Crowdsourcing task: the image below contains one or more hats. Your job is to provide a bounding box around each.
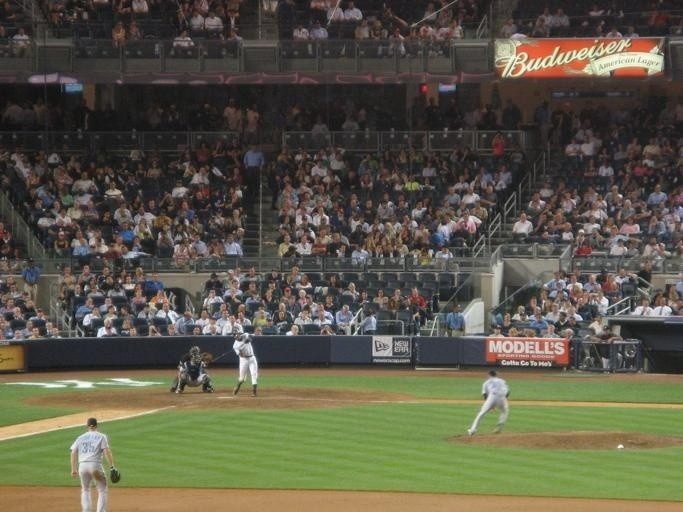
[488,370,496,376]
[87,418,97,427]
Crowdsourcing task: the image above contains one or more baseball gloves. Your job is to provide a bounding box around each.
[109,468,121,483]
[199,351,212,364]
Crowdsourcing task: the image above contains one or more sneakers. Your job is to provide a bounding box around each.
[232,385,256,396]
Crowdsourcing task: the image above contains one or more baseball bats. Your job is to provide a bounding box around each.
[211,340,245,365]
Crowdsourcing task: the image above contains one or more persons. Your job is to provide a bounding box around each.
[230,326,259,396]
[171,345,209,391]
[176,354,214,393]
[465,369,511,435]
[69,418,121,512]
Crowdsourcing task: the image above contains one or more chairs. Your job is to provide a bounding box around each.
[60,271,472,336]
